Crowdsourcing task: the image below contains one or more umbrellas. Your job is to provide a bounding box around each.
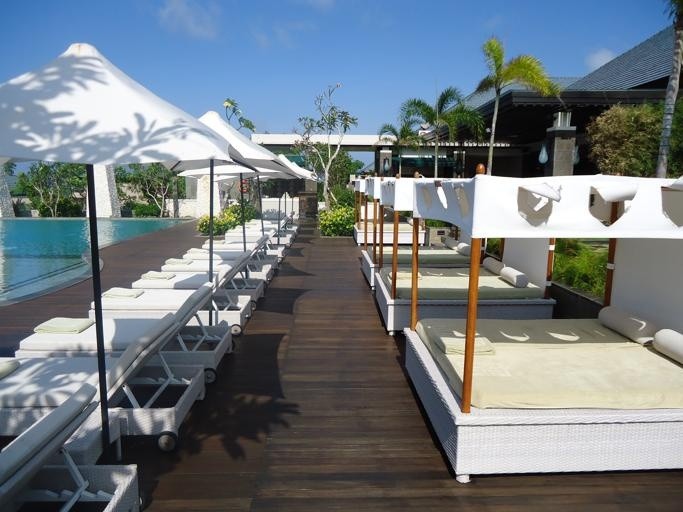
[161,111,297,281]
[0,43,258,455]
[292,161,323,214]
[252,154,312,229]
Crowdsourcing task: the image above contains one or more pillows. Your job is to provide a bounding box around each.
[652,328,682,365]
[483,257,507,275]
[597,304,655,346]
[499,267,529,288]
[457,242,471,256]
[445,237,460,250]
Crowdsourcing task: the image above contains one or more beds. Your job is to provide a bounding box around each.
[354,177,426,246]
[404,164,682,484]
[361,173,487,291]
[375,172,556,336]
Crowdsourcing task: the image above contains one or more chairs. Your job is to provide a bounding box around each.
[14,281,236,384]
[183,247,279,284]
[0,382,140,512]
[201,235,287,265]
[89,263,258,337]
[1,312,206,456]
[131,249,267,311]
[226,211,297,248]
